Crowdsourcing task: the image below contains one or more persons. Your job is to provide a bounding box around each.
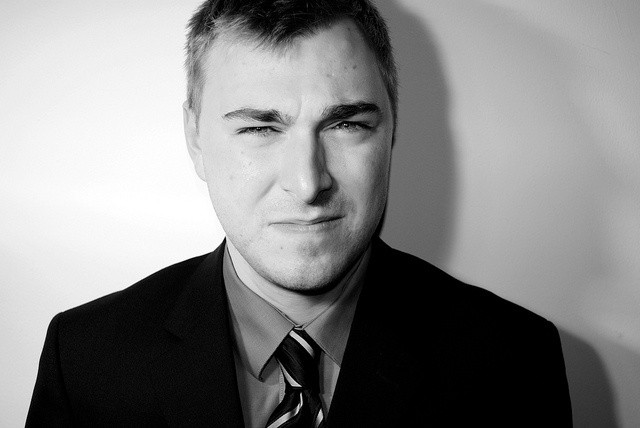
[25,3,573,427]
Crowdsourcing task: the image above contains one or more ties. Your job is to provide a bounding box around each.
[266,328,328,428]
